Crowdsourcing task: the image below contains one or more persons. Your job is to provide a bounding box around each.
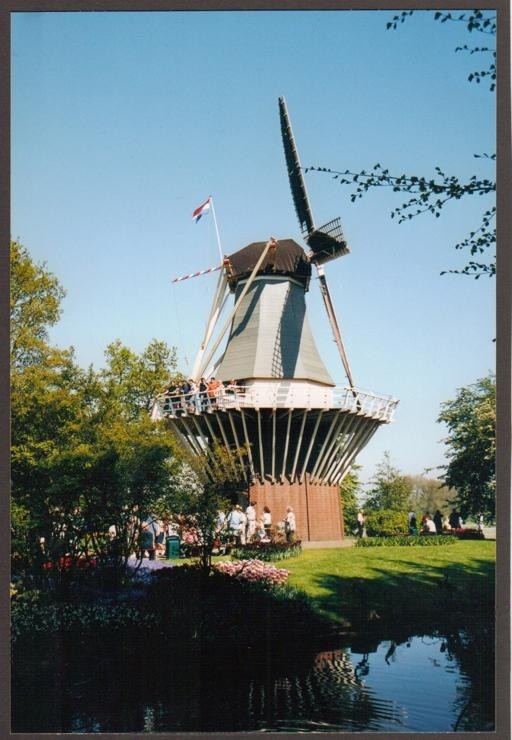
[357,507,369,539]
[408,508,419,535]
[422,512,431,521]
[98,500,299,561]
[423,516,436,535]
[432,509,444,533]
[475,511,484,530]
[448,508,463,530]
[441,515,451,530]
[159,378,245,417]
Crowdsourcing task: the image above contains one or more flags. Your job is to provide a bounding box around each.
[191,196,212,224]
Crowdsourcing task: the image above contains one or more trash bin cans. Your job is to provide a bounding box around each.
[166,535,180,560]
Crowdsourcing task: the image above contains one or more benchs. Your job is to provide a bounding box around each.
[386,533,411,536]
[127,548,159,559]
